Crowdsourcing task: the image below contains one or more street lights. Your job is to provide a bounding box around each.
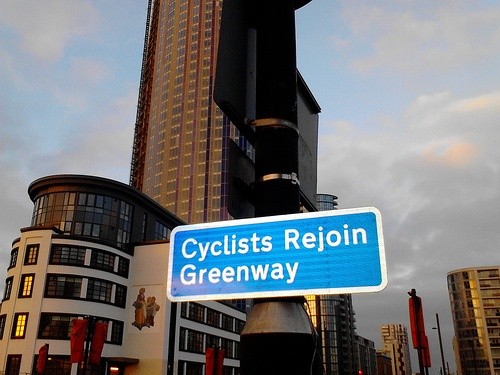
[70,314,108,375]
[203,343,224,375]
[432,313,451,375]
[407,288,431,375]
